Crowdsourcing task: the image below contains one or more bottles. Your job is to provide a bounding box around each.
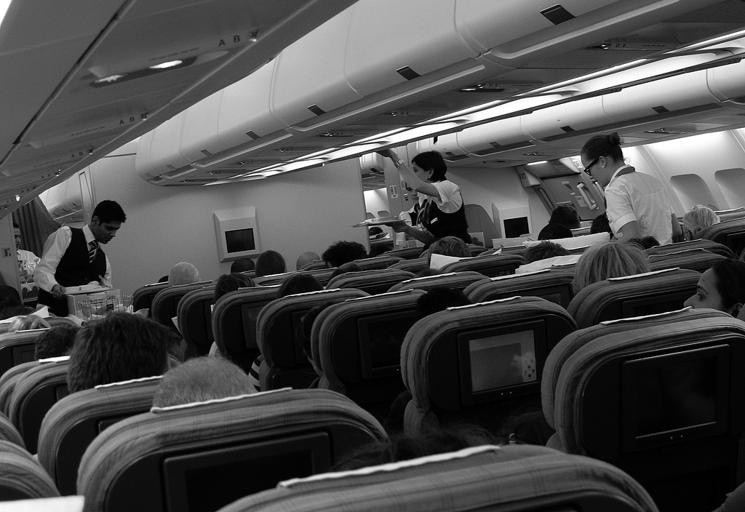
[75,296,119,320]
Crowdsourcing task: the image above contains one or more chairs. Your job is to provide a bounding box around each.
[536,304,744,508]
[209,283,283,375]
[239,284,371,392]
[304,287,433,435]
[73,386,403,512]
[165,288,221,367]
[214,440,659,512]
[2,306,92,502]
[398,295,575,451]
[150,281,202,329]
[227,210,745,330]
[128,276,175,310]
[36,374,181,499]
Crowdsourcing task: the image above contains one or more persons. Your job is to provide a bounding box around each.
[521,201,744,322]
[579,128,686,249]
[14,223,42,278]
[31,197,126,318]
[373,143,472,259]
[1,239,507,475]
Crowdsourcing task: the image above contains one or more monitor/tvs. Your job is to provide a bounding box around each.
[225,228,256,253]
[621,344,732,438]
[241,302,266,349]
[164,432,333,512]
[291,309,312,369]
[458,319,548,406]
[356,310,417,379]
[15,346,34,365]
[504,217,529,238]
[495,286,569,309]
[622,291,697,318]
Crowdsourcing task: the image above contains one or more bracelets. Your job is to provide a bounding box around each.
[393,158,406,170]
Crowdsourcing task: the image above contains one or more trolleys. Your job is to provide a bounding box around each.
[65,281,135,329]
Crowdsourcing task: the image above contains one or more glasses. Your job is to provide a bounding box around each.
[584,160,598,176]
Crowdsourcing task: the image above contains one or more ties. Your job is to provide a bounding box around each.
[88,240,99,264]
